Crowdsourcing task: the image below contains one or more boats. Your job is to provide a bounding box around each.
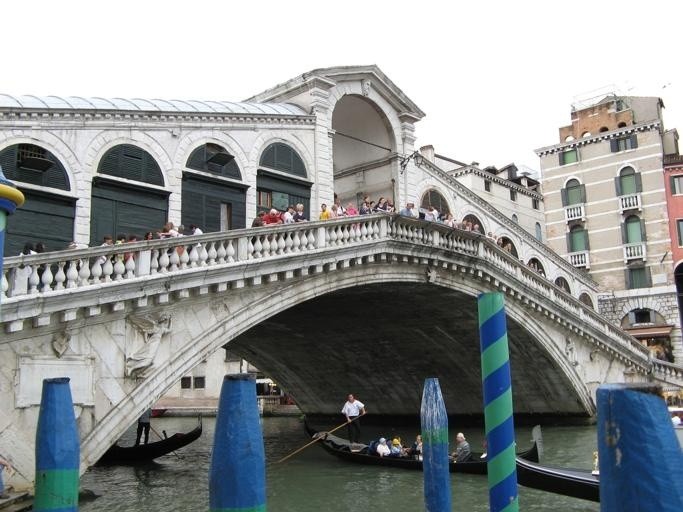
[514,453,600,504]
[303,414,545,473]
[93,413,203,465]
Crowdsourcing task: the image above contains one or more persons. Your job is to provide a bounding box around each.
[247,194,546,278]
[340,392,366,444]
[449,432,471,463]
[363,432,422,465]
[21,221,203,282]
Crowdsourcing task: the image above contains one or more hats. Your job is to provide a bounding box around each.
[379,438,386,443]
[392,439,400,445]
